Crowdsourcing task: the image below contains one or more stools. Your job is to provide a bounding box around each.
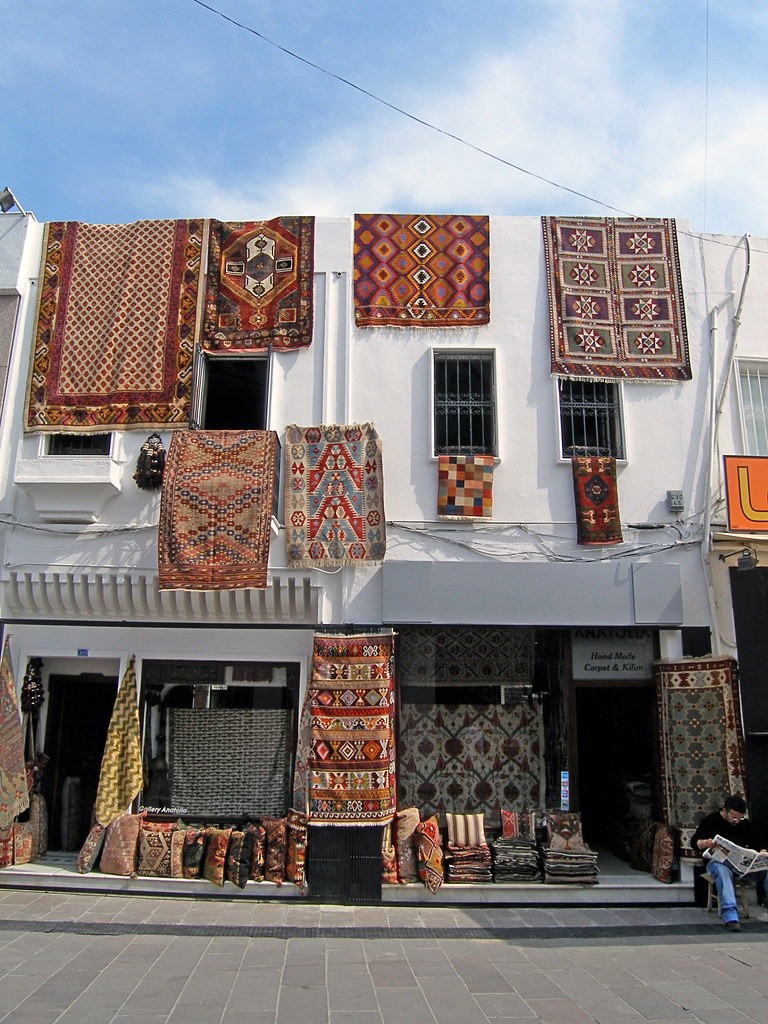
[701,872,747,918]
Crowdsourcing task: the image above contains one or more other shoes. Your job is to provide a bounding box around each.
[726,921,742,931]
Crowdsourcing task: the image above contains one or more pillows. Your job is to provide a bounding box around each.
[546,813,583,849]
[76,806,308,891]
[628,816,656,873]
[380,822,399,885]
[500,809,536,841]
[651,824,673,884]
[444,811,487,846]
[416,813,443,895]
[28,793,49,855]
[395,805,418,885]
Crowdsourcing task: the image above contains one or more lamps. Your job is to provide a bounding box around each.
[718,547,759,572]
[0,186,27,216]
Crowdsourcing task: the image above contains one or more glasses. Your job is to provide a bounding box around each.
[727,812,746,822]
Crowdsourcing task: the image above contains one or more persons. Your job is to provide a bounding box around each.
[690,795,768,931]
